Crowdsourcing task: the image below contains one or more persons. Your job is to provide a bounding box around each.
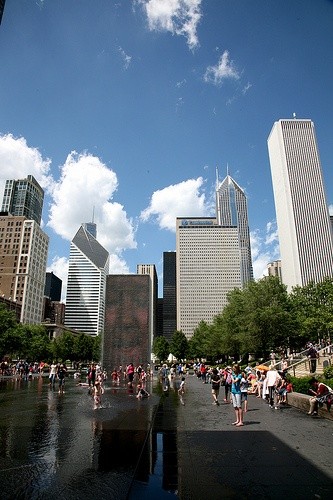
[0,337,333,426]
[210,368,220,406]
